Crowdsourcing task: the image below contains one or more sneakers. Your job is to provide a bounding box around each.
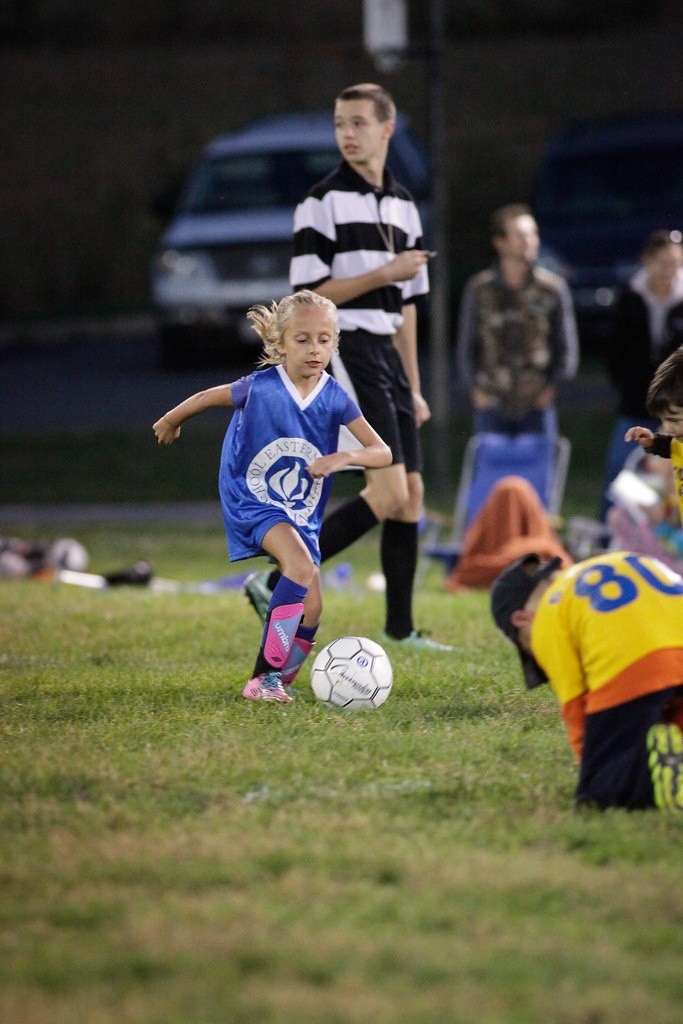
[243,671,294,705]
[242,568,280,624]
[382,628,454,653]
[645,721,683,815]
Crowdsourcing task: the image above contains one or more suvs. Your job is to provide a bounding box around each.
[148,116,430,376]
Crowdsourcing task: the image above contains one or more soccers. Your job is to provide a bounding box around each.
[309,635,395,710]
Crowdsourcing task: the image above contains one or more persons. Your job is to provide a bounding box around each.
[487,549,680,817]
[423,200,582,581]
[582,229,683,558]
[150,289,395,704]
[243,82,460,655]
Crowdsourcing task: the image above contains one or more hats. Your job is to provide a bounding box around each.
[491,552,563,690]
